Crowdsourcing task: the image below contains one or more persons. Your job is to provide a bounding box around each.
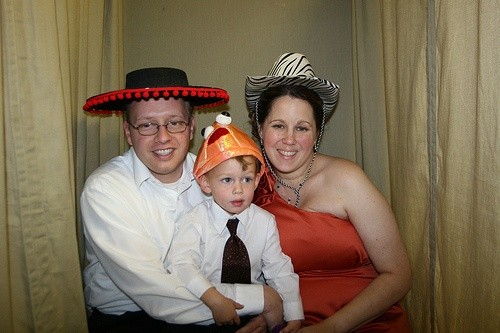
[244,53,414,333]
[79,67,284,333]
[162,112,305,333]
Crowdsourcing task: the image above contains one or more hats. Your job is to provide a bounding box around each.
[82,67,229,116]
[193,112,265,177]
[245,53,339,125]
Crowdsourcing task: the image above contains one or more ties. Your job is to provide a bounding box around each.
[220,217,252,329]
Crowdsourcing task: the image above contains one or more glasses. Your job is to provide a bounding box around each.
[124,110,193,135]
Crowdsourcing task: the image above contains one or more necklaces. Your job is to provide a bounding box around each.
[275,183,302,203]
